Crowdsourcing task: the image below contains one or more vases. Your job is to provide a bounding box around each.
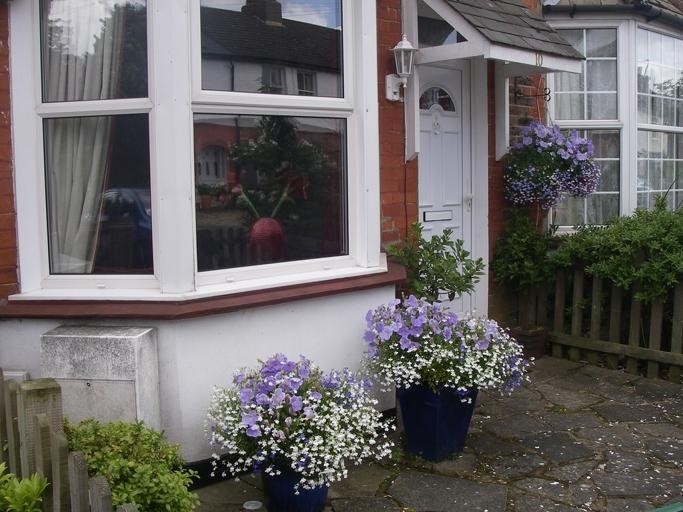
[392,379,480,465]
[246,218,286,263]
[259,454,331,512]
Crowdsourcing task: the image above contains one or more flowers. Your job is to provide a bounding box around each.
[359,294,536,404]
[226,117,332,215]
[500,122,600,210]
[203,351,396,496]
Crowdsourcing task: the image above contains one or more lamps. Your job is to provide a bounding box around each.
[384,33,420,102]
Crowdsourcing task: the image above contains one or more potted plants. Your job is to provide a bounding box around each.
[196,184,221,212]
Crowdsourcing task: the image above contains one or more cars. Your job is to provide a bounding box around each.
[635,176,654,205]
[77,185,215,260]
